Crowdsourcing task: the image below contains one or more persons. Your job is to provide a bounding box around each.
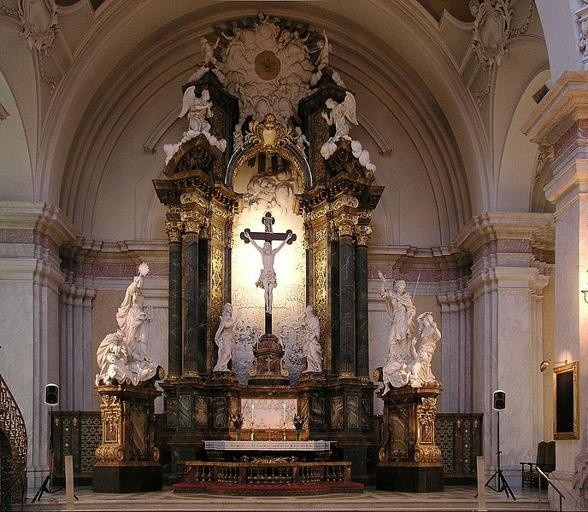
[210,301,240,373]
[242,230,294,316]
[178,10,360,151]
[376,270,441,396]
[93,261,154,388]
[298,304,323,374]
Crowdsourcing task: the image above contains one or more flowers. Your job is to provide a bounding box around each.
[293,413,304,441]
[231,415,244,441]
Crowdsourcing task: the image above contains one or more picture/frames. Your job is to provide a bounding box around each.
[552,361,580,440]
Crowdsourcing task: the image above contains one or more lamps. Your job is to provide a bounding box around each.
[540,359,567,372]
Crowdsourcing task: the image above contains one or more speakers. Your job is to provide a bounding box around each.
[44,383,59,406]
[492,390,506,412]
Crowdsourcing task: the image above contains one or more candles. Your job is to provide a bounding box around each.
[250,405,256,422]
[282,402,285,422]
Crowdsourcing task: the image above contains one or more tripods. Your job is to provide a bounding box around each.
[31,407,78,503]
[474,412,516,501]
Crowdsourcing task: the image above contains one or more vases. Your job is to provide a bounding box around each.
[296,430,302,441]
[236,428,242,441]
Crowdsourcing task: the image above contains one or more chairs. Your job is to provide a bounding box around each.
[520,441,556,489]
